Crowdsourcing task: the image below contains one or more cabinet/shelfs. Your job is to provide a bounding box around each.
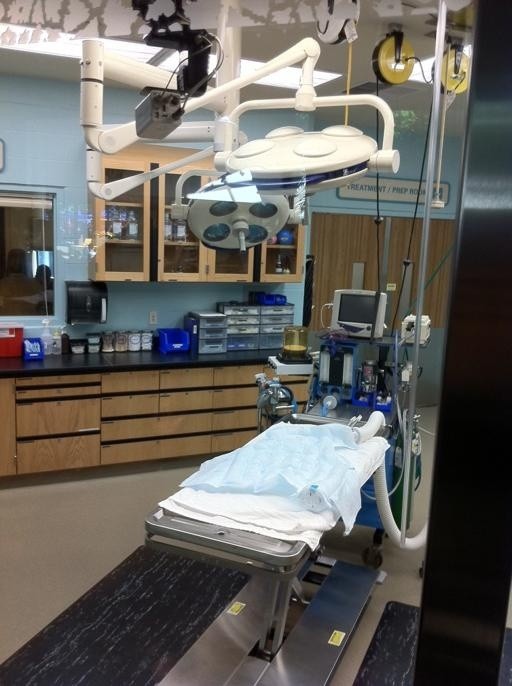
[158,162,255,284]
[16,368,103,489]
[218,303,261,353]
[1,371,17,489]
[215,358,312,456]
[189,309,228,354]
[88,160,154,283]
[256,210,304,285]
[261,303,295,351]
[102,362,215,478]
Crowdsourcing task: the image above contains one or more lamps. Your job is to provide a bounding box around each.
[188,179,289,251]
[228,124,377,193]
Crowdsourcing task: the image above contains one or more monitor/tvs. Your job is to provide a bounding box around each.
[330,288,387,339]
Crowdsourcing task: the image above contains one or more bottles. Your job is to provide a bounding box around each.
[361,359,388,399]
[126,210,138,239]
[276,255,282,273]
[323,392,341,409]
[42,320,153,355]
[112,211,122,239]
[165,213,187,240]
